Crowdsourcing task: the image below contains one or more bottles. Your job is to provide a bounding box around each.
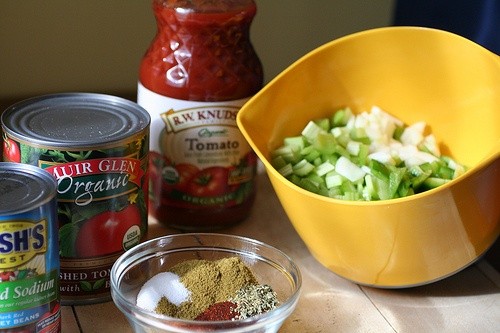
[137,0,264,233]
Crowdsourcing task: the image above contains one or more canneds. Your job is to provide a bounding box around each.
[0,161,63,333]
[0,92,151,305]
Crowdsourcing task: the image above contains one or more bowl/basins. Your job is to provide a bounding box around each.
[110,233,302,333]
[237,26,500,288]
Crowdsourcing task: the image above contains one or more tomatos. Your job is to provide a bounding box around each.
[2,133,21,163]
[145,151,257,203]
[76,205,142,262]
